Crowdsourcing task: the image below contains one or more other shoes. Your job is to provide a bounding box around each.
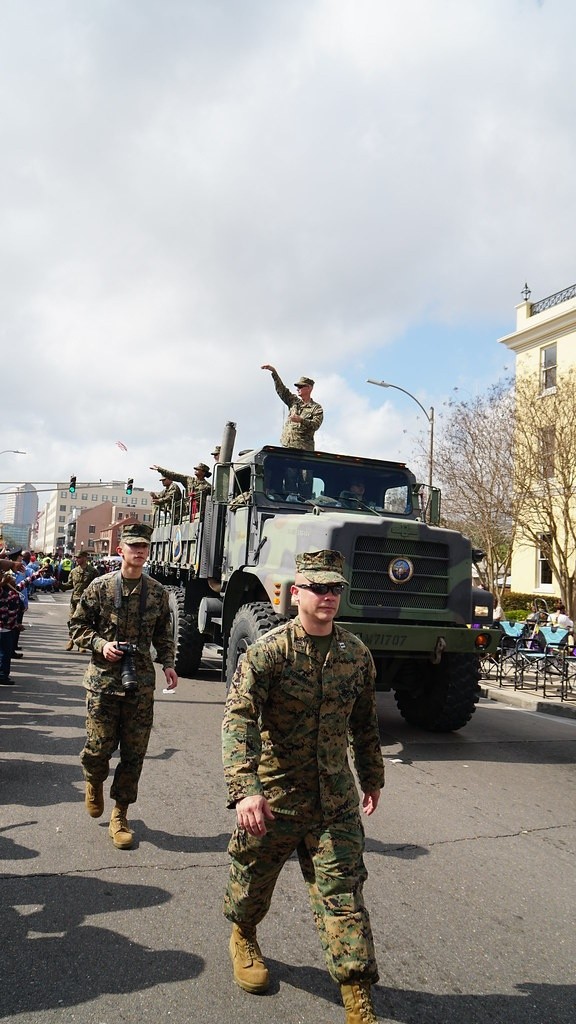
[65,641,73,650]
[15,647,22,650]
[12,653,23,658]
[0,676,15,685]
[78,647,87,652]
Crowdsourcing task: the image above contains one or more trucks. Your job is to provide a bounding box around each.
[132,423,502,733]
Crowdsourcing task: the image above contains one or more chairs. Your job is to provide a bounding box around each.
[479,620,576,703]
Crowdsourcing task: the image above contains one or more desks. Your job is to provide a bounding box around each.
[526,653,576,691]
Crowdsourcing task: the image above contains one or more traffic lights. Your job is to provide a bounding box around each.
[69,476,77,494]
[126,478,134,496]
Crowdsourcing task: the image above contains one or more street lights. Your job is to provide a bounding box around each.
[365,378,436,487]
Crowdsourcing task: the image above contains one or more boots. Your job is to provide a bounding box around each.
[108,801,133,848]
[229,921,269,993]
[86,782,104,818]
[340,979,380,1024]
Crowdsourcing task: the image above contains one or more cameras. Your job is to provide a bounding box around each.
[113,643,141,693]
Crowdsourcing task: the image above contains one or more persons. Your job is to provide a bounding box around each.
[65,550,99,652]
[221,549,385,1024]
[88,559,122,576]
[0,547,78,684]
[149,463,212,512]
[350,478,376,508]
[211,446,221,461]
[472,578,504,629]
[527,604,575,656]
[149,476,186,525]
[71,524,177,847]
[261,364,323,490]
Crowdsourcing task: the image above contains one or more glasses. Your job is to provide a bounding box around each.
[297,584,344,596]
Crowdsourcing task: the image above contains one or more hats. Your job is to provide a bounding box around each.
[295,549,350,586]
[6,546,24,559]
[121,524,153,545]
[294,376,315,386]
[74,549,87,558]
[211,446,221,455]
[159,475,166,481]
[194,462,210,472]
[30,556,36,562]
